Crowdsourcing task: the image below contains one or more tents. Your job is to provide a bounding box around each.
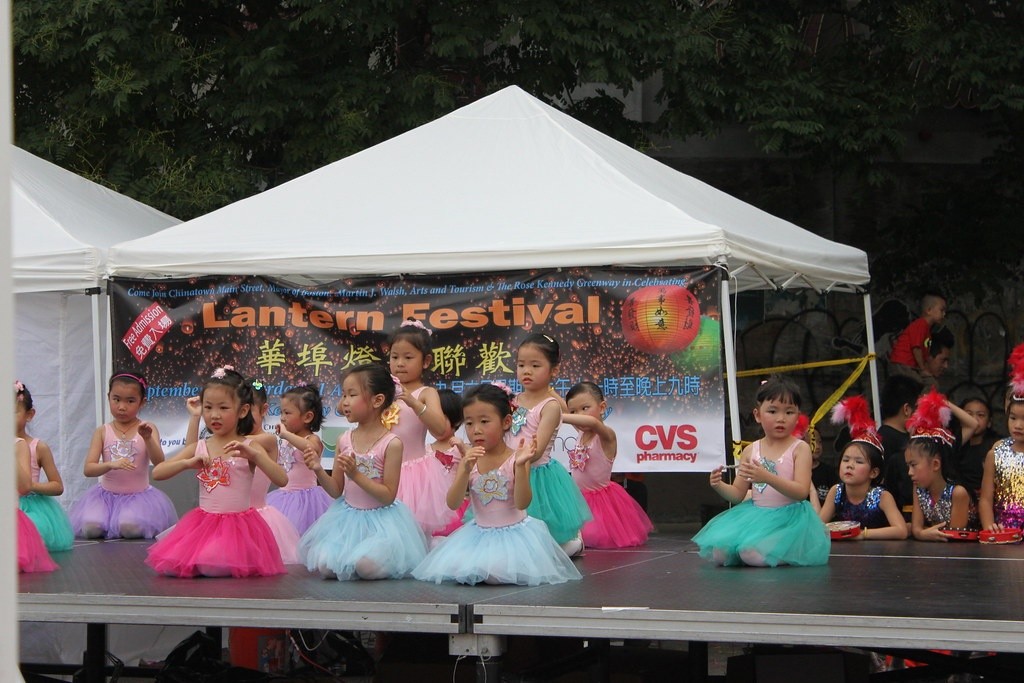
[12,84,883,483]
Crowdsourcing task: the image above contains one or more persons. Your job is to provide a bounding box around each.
[908,426,977,544]
[150,365,300,579]
[68,372,178,539]
[265,386,332,539]
[11,380,74,570]
[294,362,430,582]
[691,376,830,567]
[978,396,1024,532]
[952,397,1005,489]
[815,439,909,542]
[411,383,583,588]
[384,316,460,537]
[503,333,594,557]
[427,389,471,537]
[548,381,654,549]
[876,374,979,505]
[890,295,954,391]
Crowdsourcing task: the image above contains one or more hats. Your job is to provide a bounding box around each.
[904,389,956,448]
[831,395,885,454]
[1005,344,1024,411]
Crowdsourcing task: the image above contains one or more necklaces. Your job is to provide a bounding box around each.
[112,420,139,439]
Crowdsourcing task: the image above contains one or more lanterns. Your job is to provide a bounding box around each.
[620,284,700,358]
[670,316,722,377]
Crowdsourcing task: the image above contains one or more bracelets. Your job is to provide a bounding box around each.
[548,387,554,393]
[418,404,426,418]
[863,527,867,538]
